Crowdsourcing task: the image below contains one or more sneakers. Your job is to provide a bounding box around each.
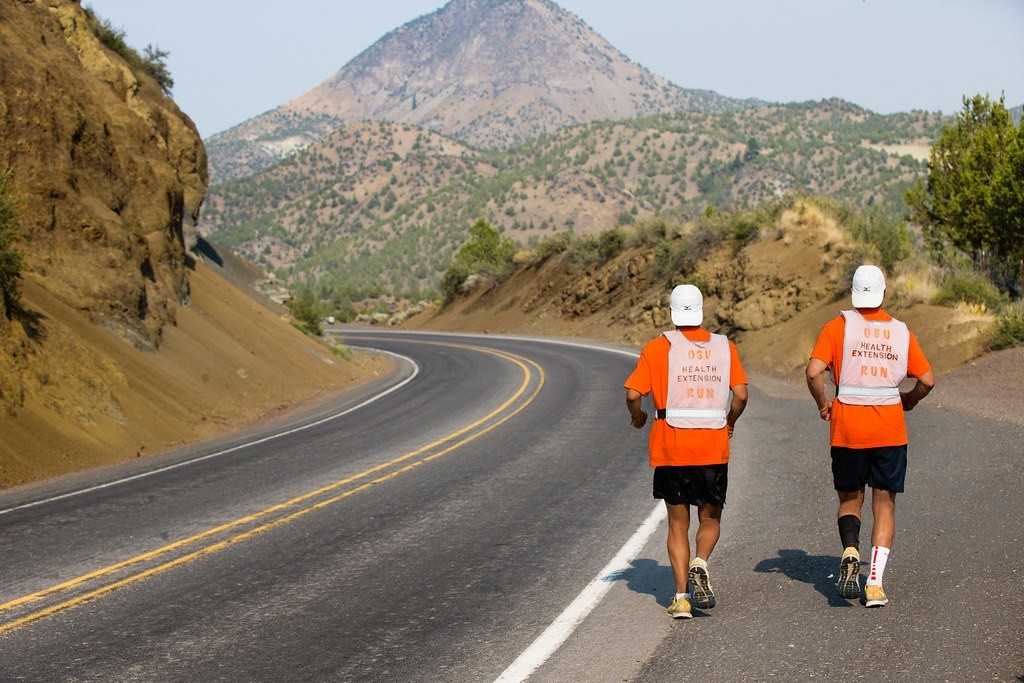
[688,559,716,609]
[837,547,861,600]
[864,584,889,607]
[666,596,693,618]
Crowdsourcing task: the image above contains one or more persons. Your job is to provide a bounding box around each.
[624,284,748,619]
[805,264,935,607]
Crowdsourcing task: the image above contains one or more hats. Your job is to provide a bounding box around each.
[670,285,703,326]
[852,265,886,308]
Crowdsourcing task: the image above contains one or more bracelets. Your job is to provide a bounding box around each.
[818,399,829,412]
[906,393,918,406]
[630,411,645,425]
